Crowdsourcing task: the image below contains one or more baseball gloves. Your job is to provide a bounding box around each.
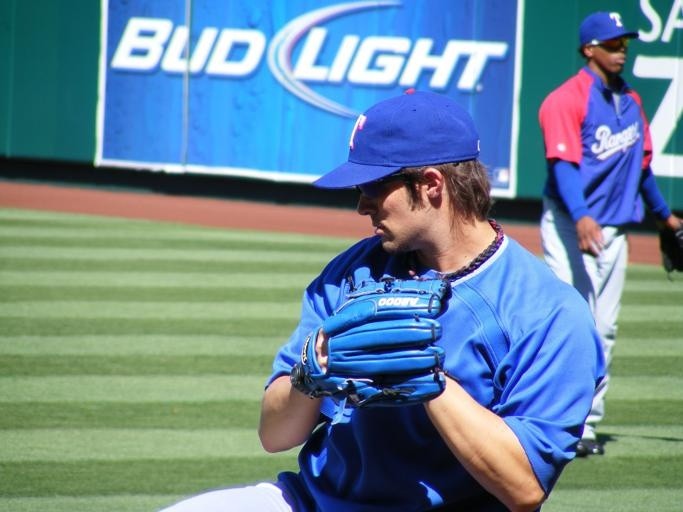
[290,269,452,408]
[657,223,683,280]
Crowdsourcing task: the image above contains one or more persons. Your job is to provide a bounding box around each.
[150,87,606,512]
[534,8,681,459]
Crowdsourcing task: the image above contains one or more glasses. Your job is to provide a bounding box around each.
[580,36,629,52]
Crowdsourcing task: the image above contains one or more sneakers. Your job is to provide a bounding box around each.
[576,438,605,458]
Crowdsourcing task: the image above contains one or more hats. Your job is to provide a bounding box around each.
[311,88,481,191]
[577,10,640,49]
